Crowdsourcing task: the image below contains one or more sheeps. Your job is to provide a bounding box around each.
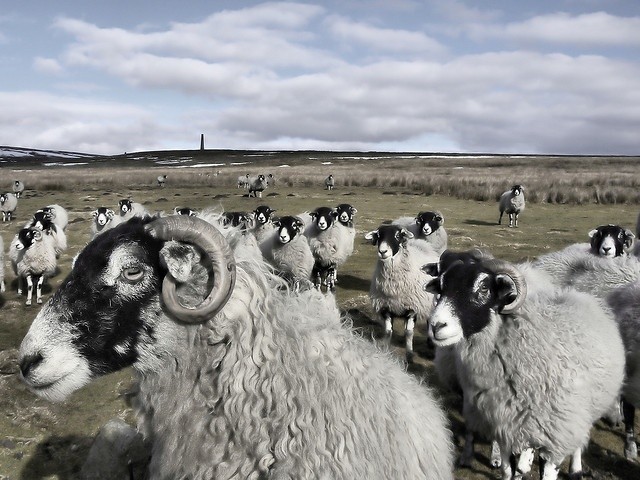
[157,175,167,188]
[18,211,456,479]
[266,173,275,188]
[12,181,24,199]
[531,223,639,299]
[0,192,17,223]
[0,235,6,294]
[89,206,125,234]
[322,204,357,288]
[499,185,525,228]
[249,205,279,260]
[216,210,256,237]
[390,210,446,259]
[420,249,502,467]
[248,175,268,199]
[117,199,148,218]
[257,215,315,291]
[9,227,57,306]
[605,281,639,455]
[296,207,339,294]
[364,223,437,363]
[423,260,627,479]
[237,173,249,189]
[23,212,67,258]
[325,175,334,190]
[36,203,68,232]
